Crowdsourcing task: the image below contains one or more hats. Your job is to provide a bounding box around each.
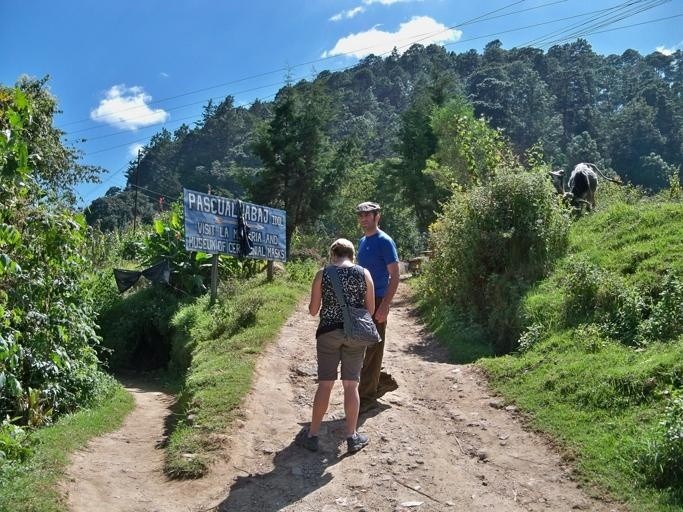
[356,201,381,212]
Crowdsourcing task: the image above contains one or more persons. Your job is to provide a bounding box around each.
[354,201,400,414]
[294,238,376,453]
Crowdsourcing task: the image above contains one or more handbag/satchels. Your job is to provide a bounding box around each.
[343,307,382,345]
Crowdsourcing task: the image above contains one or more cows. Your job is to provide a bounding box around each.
[565,163,623,223]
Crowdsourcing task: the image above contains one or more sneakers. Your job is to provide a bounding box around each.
[295,426,318,451]
[377,377,399,399]
[347,432,369,453]
[360,399,377,413]
